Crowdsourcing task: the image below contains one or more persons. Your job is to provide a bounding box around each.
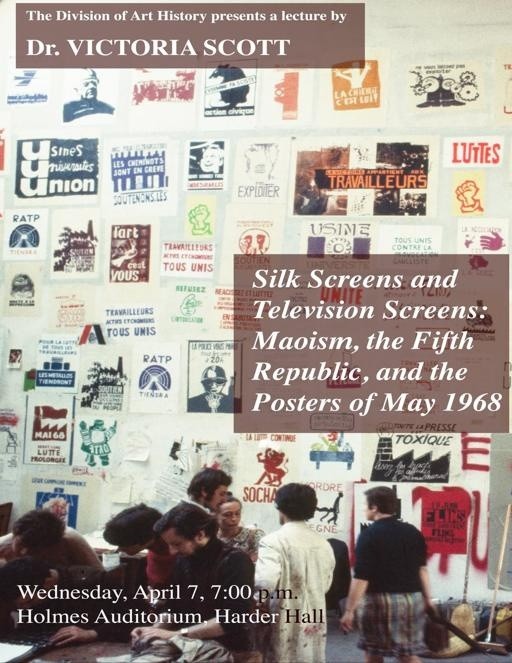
[64,68,114,122]
[188,365,241,412]
[340,487,437,663]
[255,483,336,663]
[0,467,266,649]
[200,144,223,173]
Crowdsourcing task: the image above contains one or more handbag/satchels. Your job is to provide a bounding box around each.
[152,639,235,663]
[423,620,449,651]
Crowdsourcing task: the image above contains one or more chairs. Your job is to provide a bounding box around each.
[325,539,351,635]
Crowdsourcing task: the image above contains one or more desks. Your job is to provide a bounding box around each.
[24,640,264,663]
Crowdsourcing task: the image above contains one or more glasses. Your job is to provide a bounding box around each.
[204,378,228,384]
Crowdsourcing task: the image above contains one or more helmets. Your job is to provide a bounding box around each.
[201,366,227,381]
